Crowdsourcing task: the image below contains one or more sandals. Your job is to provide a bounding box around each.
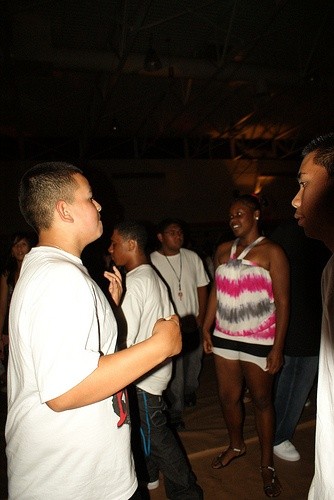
[260,463,283,498]
[211,443,247,470]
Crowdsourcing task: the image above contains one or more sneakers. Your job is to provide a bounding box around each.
[273,439,301,463]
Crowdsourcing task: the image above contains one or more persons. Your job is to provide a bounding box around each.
[203,193,289,498]
[0,135,334,500]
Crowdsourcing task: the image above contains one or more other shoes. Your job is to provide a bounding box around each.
[147,480,160,490]
[185,393,197,406]
[170,413,185,432]
